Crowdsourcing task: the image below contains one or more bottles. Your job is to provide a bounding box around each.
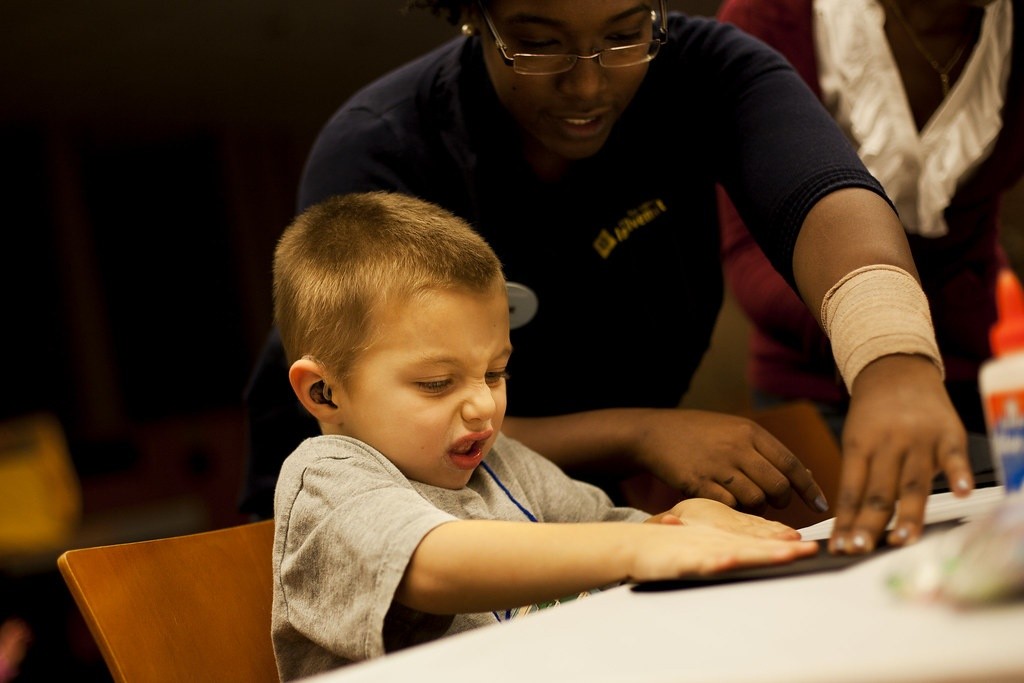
[975,268,1024,497]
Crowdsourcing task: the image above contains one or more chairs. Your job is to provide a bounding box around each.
[55,518,325,683]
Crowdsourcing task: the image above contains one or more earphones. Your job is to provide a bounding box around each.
[310,381,338,407]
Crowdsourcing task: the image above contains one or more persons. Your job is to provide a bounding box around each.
[707,0,1024,491]
[238,1,972,556]
[272,193,819,683]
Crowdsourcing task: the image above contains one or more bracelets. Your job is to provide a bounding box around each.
[820,265,946,398]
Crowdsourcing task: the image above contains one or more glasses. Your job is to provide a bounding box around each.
[474,1,669,76]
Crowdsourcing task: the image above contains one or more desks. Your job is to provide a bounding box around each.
[268,485,1023,679]
